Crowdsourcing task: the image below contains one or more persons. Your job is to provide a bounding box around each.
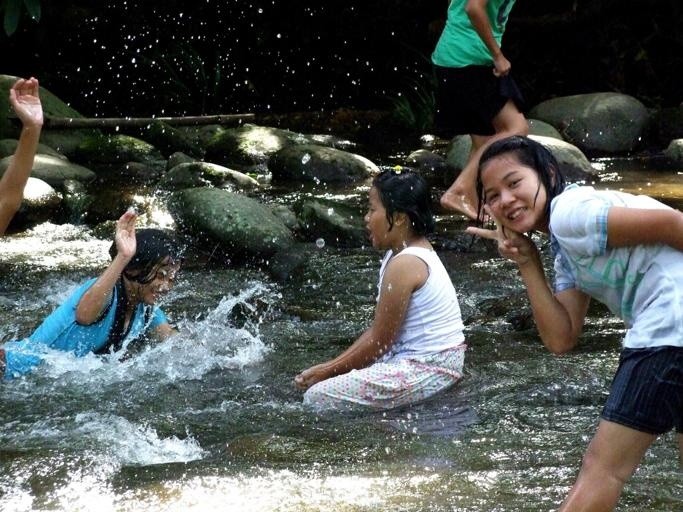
[466,134,683,512]
[294,169,468,421]
[0,76,44,236]
[431,0,529,222]
[0,209,188,383]
